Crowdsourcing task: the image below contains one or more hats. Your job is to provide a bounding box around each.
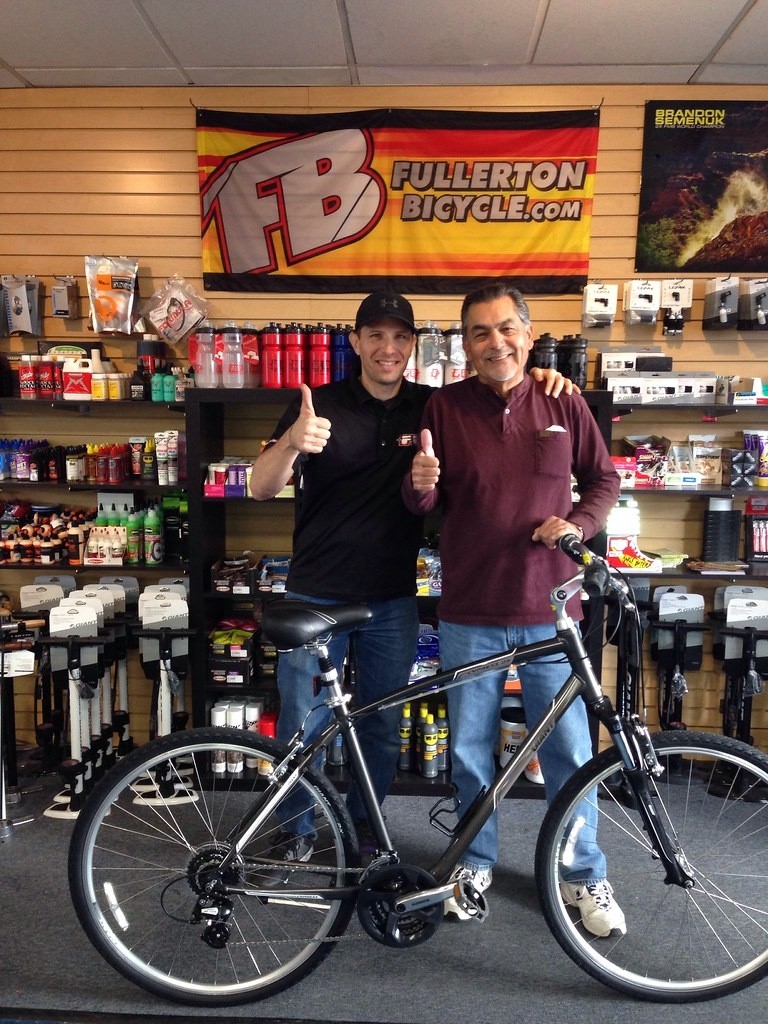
[355,293,417,333]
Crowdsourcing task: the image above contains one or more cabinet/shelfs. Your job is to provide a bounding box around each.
[182,385,614,800]
[608,398,768,580]
[0,394,185,575]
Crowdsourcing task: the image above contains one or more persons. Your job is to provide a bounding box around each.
[249,290,582,890]
[400,283,627,937]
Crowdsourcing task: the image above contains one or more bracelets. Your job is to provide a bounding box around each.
[575,524,584,542]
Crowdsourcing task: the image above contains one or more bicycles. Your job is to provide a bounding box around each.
[68,519,768,1009]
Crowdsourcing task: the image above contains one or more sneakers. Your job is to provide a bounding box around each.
[250,832,313,889]
[558,881,626,937]
[444,866,493,920]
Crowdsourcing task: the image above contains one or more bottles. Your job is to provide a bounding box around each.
[0,318,589,789]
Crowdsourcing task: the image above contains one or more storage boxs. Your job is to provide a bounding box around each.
[202,480,444,690]
